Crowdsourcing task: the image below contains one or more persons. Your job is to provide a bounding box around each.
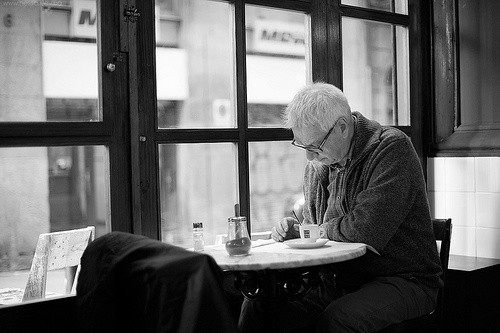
[238,82,446,333]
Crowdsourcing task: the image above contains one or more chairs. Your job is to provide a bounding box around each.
[67,232,208,333]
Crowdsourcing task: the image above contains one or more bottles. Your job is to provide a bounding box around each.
[225,217,250,256]
[192,222,205,252]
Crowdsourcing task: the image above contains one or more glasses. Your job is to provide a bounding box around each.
[291,117,347,153]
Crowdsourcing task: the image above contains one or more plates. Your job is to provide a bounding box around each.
[283,238,329,248]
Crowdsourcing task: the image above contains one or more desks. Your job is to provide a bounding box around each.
[180,235,368,333]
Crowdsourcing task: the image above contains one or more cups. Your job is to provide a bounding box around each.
[299,225,325,239]
